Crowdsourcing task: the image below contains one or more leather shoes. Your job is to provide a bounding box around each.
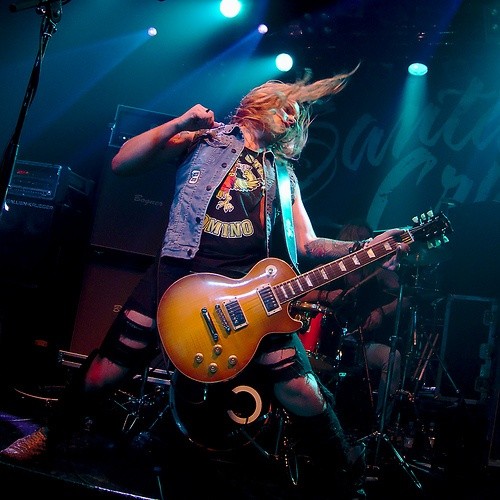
[0,426,47,460]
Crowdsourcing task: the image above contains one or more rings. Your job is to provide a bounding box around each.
[206,109,211,113]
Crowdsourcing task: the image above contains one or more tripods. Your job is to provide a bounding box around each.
[349,251,421,490]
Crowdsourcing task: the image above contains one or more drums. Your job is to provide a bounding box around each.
[288,301,347,374]
[170,370,270,451]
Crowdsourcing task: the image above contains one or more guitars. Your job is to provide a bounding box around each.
[156,209,456,384]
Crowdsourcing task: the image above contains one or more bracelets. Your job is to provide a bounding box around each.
[378,305,387,322]
[349,239,366,256]
[325,290,331,303]
[317,290,322,302]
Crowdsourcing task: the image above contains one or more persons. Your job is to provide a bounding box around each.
[0,61,411,500]
[308,219,414,448]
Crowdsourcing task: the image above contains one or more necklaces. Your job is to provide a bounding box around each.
[234,134,271,164]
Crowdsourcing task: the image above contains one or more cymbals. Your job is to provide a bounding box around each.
[383,287,439,299]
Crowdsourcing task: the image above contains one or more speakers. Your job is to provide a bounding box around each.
[90,146,183,258]
[414,294,499,405]
